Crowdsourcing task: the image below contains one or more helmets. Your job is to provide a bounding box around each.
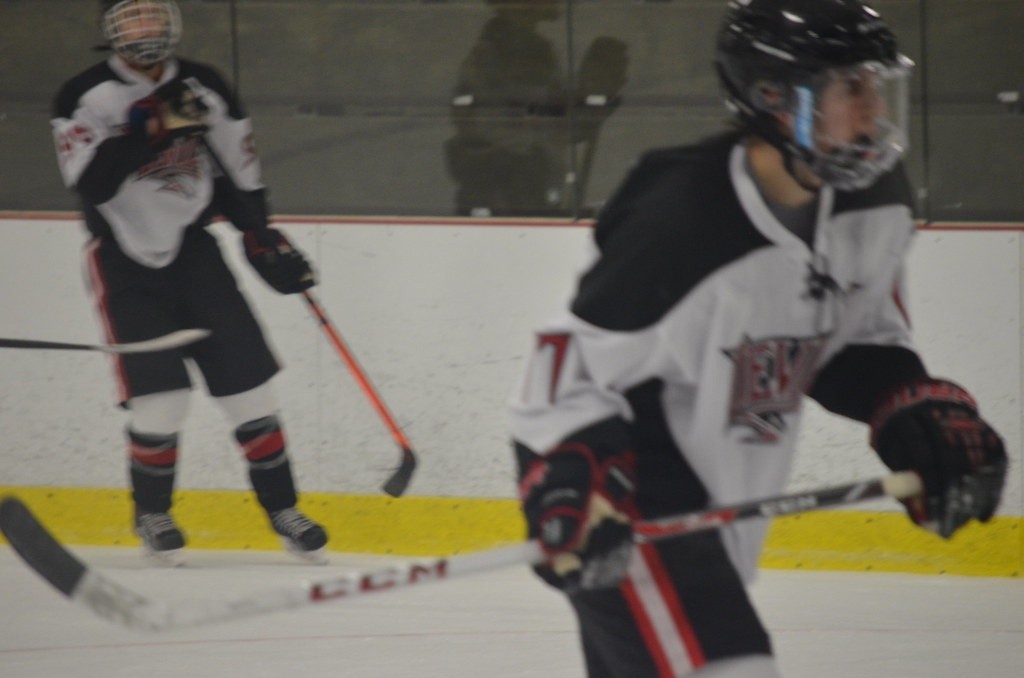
[716,0,900,191]
[93,0,182,70]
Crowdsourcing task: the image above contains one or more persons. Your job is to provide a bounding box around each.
[48,0,330,553]
[444,0,630,219]
[509,0,1011,678]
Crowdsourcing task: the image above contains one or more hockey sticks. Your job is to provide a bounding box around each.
[0,328,215,354]
[197,133,418,499]
[571,133,594,218]
[0,472,925,636]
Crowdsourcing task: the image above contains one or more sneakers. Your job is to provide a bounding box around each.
[134,505,186,569]
[268,508,331,567]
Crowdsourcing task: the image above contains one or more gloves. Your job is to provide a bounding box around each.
[870,378,1008,542]
[129,80,212,151]
[514,420,639,599]
[241,224,318,294]
